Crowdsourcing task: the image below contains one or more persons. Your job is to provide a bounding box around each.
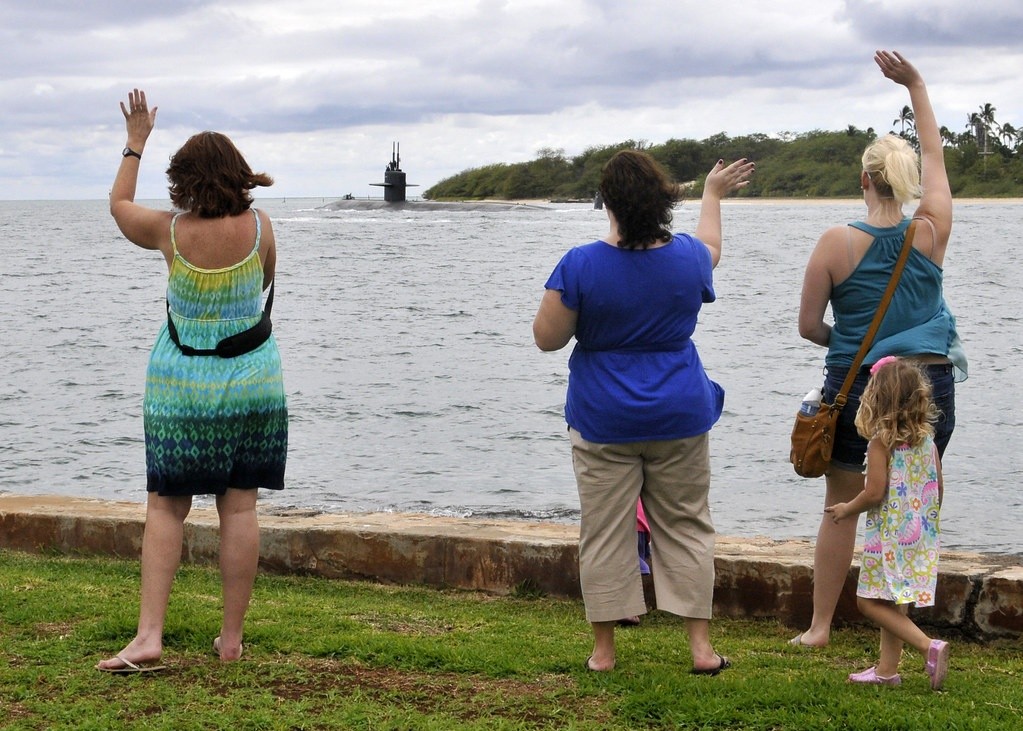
[616,494,650,626]
[823,355,951,691]
[786,50,956,668]
[93,87,289,673]
[532,148,756,677]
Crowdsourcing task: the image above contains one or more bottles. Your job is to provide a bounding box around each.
[801,387,823,417]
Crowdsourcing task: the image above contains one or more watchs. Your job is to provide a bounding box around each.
[122,146,142,160]
